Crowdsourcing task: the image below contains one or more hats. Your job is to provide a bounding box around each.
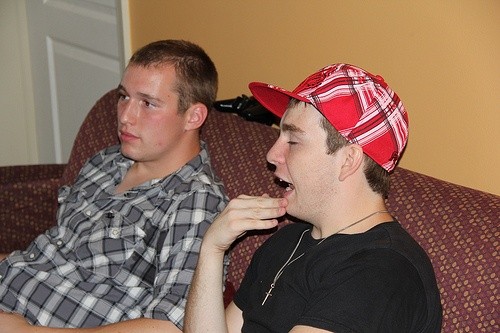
[247,62,411,172]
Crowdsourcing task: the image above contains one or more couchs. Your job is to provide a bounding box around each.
[0,86,500,333]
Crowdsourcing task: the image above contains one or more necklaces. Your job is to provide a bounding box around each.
[261,210,389,306]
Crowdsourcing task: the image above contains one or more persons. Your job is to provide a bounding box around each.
[0,39,230,333]
[183,63,442,333]
